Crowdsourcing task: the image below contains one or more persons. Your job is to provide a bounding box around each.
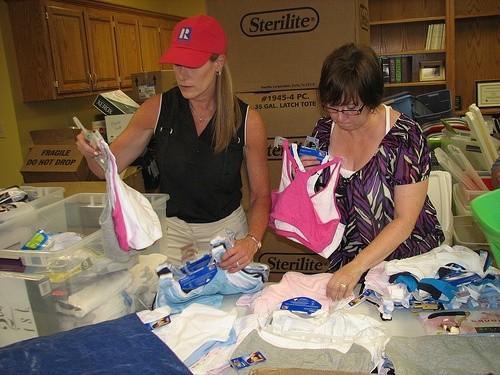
[292,43,445,302]
[76,14,271,273]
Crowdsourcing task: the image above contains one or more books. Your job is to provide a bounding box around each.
[377,54,412,84]
[425,24,446,50]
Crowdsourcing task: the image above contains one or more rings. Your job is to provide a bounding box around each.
[341,283,348,287]
[237,261,240,267]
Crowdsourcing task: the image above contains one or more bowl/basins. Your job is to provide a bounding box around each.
[468,187,500,270]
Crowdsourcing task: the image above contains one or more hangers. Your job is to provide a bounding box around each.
[72,114,109,155]
[273,129,345,170]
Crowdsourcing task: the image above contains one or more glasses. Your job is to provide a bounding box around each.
[320,102,366,116]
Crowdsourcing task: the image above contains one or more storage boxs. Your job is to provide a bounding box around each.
[0,69,176,347]
[203,0,500,281]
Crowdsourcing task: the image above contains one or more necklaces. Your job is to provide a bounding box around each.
[190,104,203,122]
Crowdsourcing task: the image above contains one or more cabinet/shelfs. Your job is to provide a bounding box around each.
[0,0,196,102]
[368,0,500,123]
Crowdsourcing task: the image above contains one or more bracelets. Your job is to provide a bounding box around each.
[247,234,262,249]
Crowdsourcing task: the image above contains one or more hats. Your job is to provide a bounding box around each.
[158,14,227,68]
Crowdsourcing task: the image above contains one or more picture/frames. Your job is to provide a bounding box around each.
[418,59,445,81]
